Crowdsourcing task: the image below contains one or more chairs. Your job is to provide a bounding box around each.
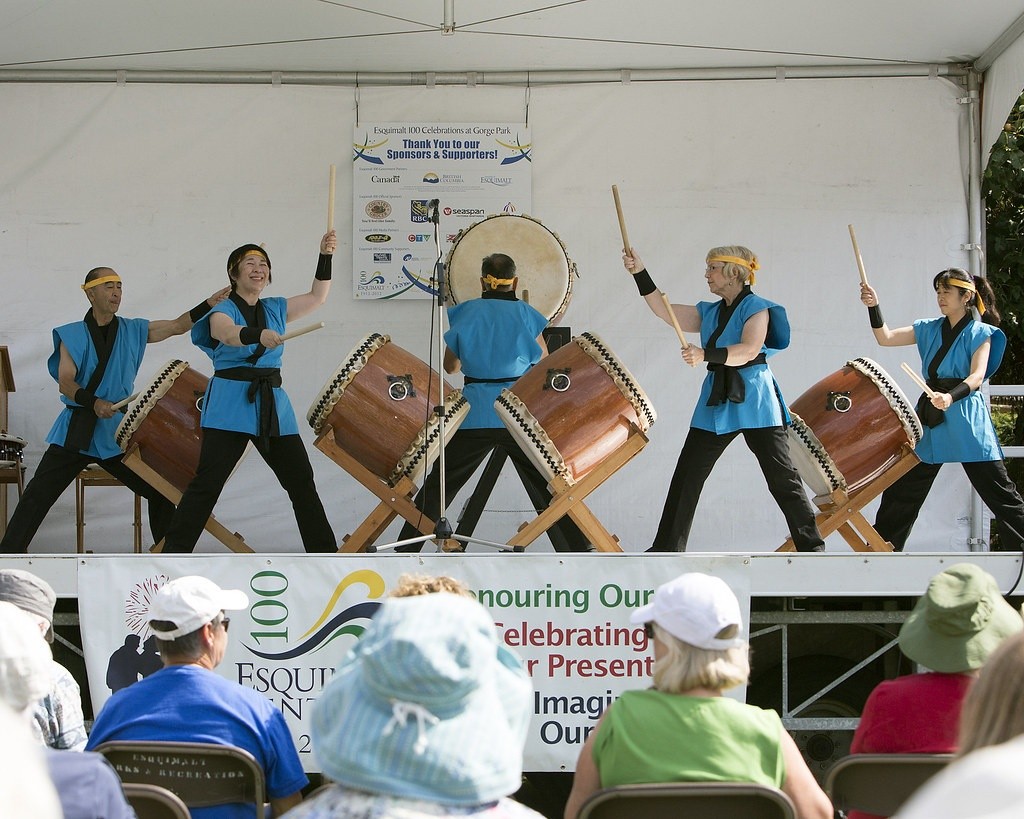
[89,740,959,818]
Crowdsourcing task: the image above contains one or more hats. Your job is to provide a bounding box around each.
[310,593,536,807]
[630,568,741,651]
[897,564,1024,673]
[0,602,53,725]
[0,568,57,644]
[146,575,250,641]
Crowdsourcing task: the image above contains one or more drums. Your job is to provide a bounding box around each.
[443,210,574,328]
[303,329,472,487]
[783,353,925,499]
[492,328,657,486]
[0,432,28,469]
[112,358,211,493]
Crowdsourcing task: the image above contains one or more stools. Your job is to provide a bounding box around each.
[75,468,142,555]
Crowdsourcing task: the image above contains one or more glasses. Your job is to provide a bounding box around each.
[645,621,656,639]
[705,265,724,273]
[215,618,230,633]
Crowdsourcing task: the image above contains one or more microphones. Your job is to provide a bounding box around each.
[427,199,440,209]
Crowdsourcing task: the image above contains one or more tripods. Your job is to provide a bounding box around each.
[366,203,526,556]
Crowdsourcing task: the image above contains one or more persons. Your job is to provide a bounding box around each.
[84,576,310,819]
[859,267,1024,552]
[564,572,833,819]
[847,563,1023,819]
[0,267,232,553]
[161,230,339,553]
[276,574,547,818]
[0,568,138,819]
[890,631,1024,819]
[394,253,598,554]
[622,246,825,553]
